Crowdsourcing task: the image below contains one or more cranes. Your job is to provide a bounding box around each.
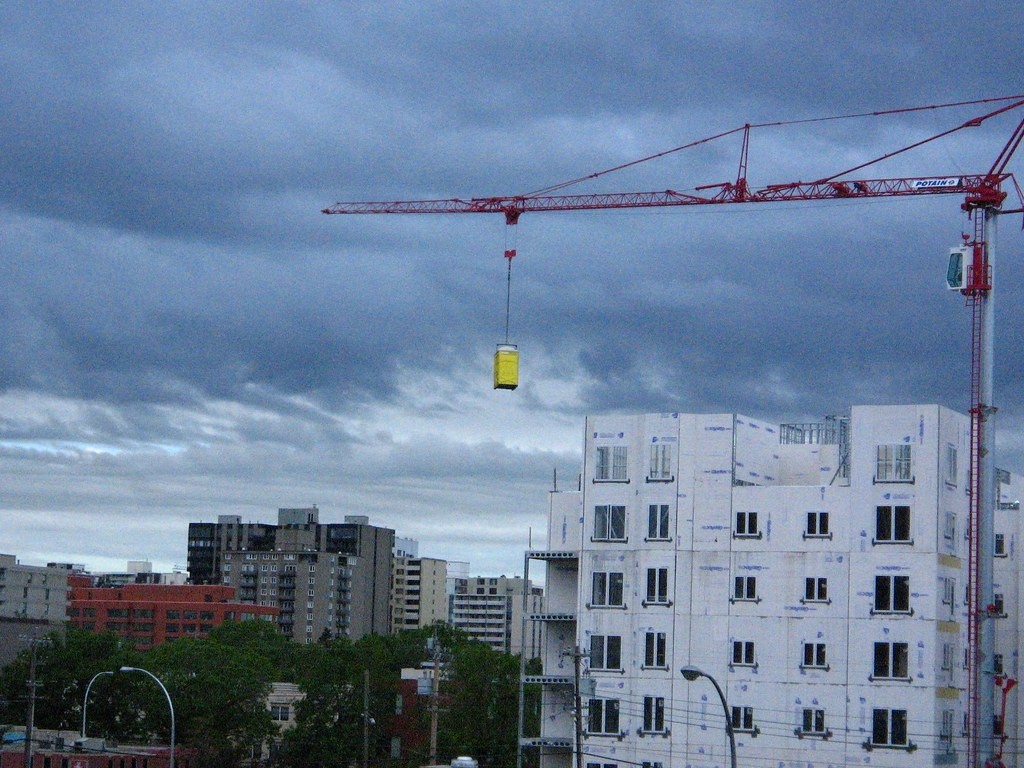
[320,90,1023,766]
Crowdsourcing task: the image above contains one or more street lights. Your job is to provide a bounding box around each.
[81,671,113,737]
[120,665,176,768]
[10,626,53,767]
[678,665,737,768]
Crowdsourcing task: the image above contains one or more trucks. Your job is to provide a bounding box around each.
[69,735,108,752]
